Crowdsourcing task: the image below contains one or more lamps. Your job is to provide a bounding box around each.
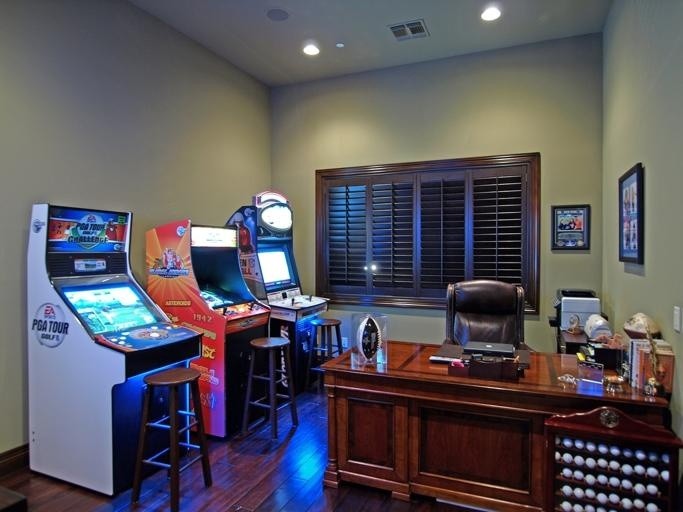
[623,312,661,396]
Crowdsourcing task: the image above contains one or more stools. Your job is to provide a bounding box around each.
[132,368,213,511]
[309,318,343,394]
[242,337,299,439]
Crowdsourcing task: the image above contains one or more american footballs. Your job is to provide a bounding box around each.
[354,317,382,361]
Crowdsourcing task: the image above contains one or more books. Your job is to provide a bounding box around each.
[429,345,464,362]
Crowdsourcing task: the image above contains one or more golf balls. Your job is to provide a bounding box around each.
[555,437,669,511]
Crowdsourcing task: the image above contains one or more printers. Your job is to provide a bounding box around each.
[549,296,601,331]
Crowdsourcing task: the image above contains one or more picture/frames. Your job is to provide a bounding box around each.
[551,205,590,250]
[617,161,645,265]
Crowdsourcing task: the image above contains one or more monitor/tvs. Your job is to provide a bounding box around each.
[258,247,291,287]
[62,282,161,335]
[199,286,235,308]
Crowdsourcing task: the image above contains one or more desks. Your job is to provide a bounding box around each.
[321,339,672,511]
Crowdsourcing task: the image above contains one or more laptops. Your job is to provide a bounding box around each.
[464,341,514,357]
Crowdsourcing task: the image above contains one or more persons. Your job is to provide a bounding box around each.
[559,216,583,230]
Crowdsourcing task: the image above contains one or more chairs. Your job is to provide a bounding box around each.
[543,404,683,510]
[442,280,533,351]
